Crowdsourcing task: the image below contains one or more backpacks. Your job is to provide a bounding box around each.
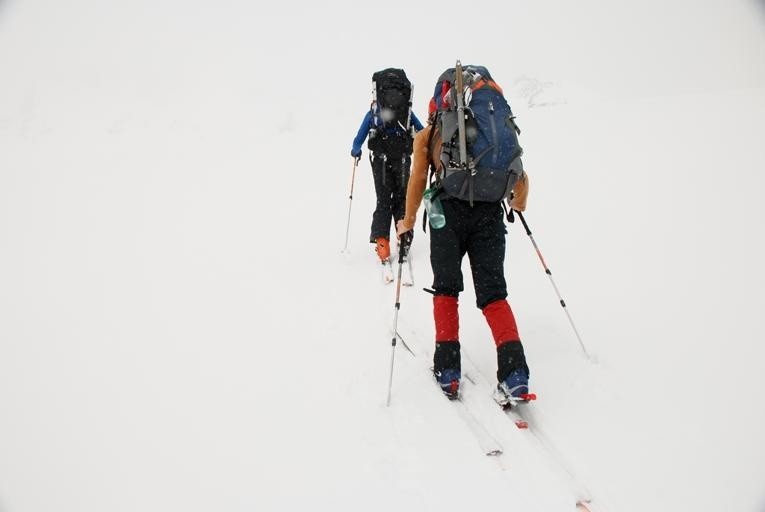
[371,68,414,160]
[433,65,523,202]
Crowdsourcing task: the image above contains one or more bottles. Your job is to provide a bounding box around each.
[423,188,448,231]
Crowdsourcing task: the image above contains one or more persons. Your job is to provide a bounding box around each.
[390,97,535,406]
[350,92,426,265]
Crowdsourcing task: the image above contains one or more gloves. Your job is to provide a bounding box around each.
[351,149,361,157]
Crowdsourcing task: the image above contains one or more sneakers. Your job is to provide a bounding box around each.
[495,370,530,405]
[436,368,461,400]
[376,237,391,264]
